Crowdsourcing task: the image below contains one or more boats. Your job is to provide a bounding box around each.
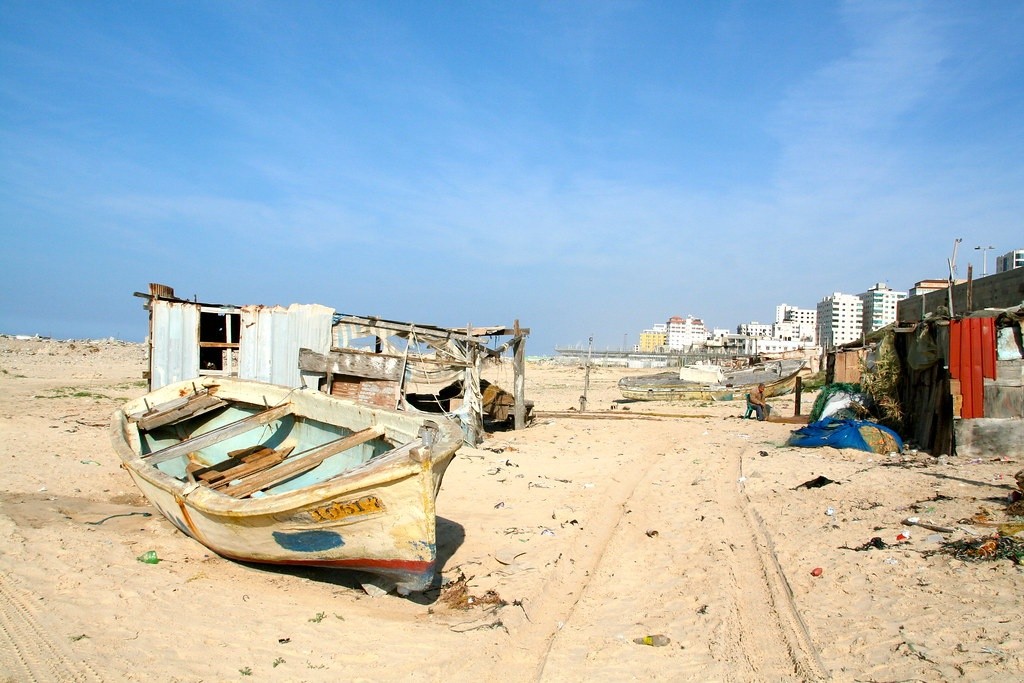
[618,357,808,401]
[785,420,899,454]
[109,375,464,592]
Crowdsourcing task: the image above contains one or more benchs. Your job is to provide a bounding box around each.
[140,403,295,466]
[220,424,386,499]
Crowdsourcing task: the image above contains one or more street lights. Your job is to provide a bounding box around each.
[975,245,995,277]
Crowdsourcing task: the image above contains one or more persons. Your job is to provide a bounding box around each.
[749,383,766,421]
